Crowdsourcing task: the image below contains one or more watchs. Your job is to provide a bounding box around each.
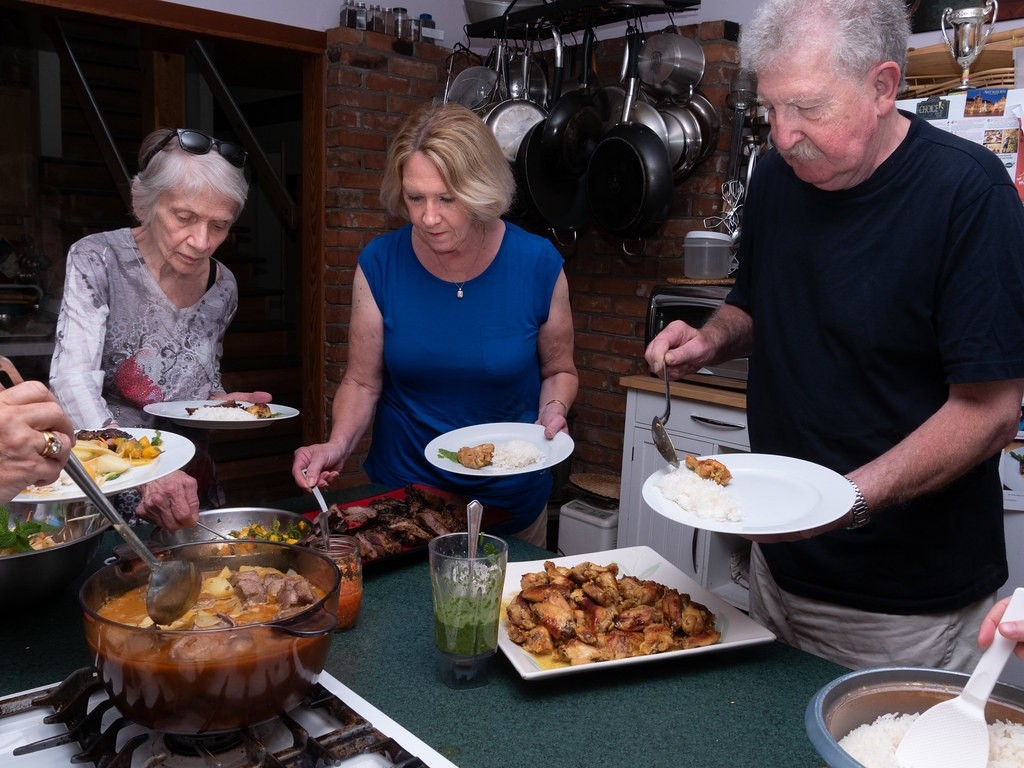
[844,476,871,530]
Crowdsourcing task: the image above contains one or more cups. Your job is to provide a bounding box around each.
[428,530,508,688]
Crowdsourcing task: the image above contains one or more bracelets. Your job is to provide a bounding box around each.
[546,400,568,418]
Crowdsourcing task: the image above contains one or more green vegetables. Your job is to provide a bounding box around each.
[230,515,302,543]
[438,449,458,463]
[0,505,55,553]
[105,429,163,480]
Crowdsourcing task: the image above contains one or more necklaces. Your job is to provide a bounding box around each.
[436,224,486,298]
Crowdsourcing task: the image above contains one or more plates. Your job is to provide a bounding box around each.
[142,396,301,426]
[638,453,860,535]
[422,421,577,478]
[8,431,194,502]
[496,545,778,679]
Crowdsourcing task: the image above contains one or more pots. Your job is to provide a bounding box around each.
[444,22,721,267]
[807,666,1024,768]
[75,538,343,735]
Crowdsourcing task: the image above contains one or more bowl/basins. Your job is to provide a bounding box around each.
[150,507,314,564]
[0,503,114,596]
[681,230,732,278]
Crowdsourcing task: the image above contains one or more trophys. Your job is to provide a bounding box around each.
[941,0,998,90]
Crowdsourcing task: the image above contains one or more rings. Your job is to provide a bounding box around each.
[40,430,62,457]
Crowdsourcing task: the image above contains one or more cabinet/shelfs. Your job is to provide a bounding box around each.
[617,388,1024,691]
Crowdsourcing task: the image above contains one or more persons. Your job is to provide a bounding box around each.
[49,129,273,531]
[645,0,1024,675]
[0,380,76,507]
[978,595,1024,660]
[292,103,579,550]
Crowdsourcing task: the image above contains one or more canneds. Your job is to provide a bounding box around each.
[311,534,363,632]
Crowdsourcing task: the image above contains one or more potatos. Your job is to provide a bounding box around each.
[201,565,285,597]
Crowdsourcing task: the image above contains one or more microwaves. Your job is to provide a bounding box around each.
[646,283,750,392]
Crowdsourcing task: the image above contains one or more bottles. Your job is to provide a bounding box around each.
[299,534,363,636]
[340,1,440,45]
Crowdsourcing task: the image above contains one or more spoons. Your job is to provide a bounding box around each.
[651,358,681,468]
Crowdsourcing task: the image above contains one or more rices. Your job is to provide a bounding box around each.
[189,406,257,421]
[492,440,549,468]
[837,712,1024,768]
[652,467,743,523]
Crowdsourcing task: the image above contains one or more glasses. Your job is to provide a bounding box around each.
[142,129,249,170]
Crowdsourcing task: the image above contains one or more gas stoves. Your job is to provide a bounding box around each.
[0,667,459,768]
